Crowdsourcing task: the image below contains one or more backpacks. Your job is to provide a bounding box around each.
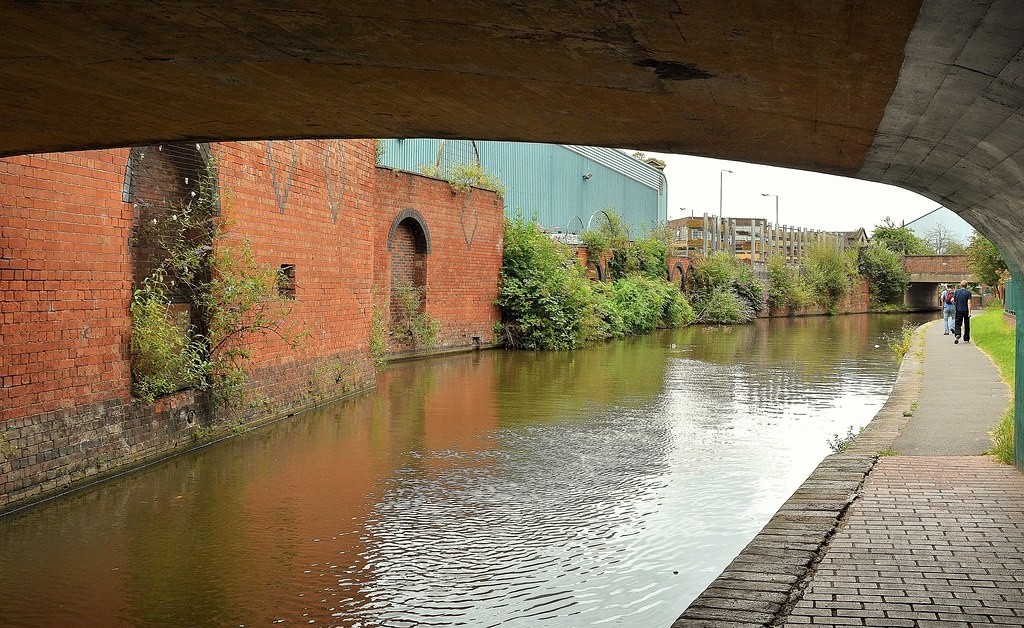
[945,289,955,305]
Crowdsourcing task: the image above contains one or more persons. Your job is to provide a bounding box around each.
[951,280,972,344]
[941,284,956,335]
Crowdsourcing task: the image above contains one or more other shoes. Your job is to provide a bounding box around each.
[964,341,969,343]
[954,335,960,344]
[950,329,955,334]
[944,332,949,335]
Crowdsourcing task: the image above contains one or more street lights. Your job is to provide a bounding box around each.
[762,194,778,226]
[720,169,733,226]
[680,207,693,240]
